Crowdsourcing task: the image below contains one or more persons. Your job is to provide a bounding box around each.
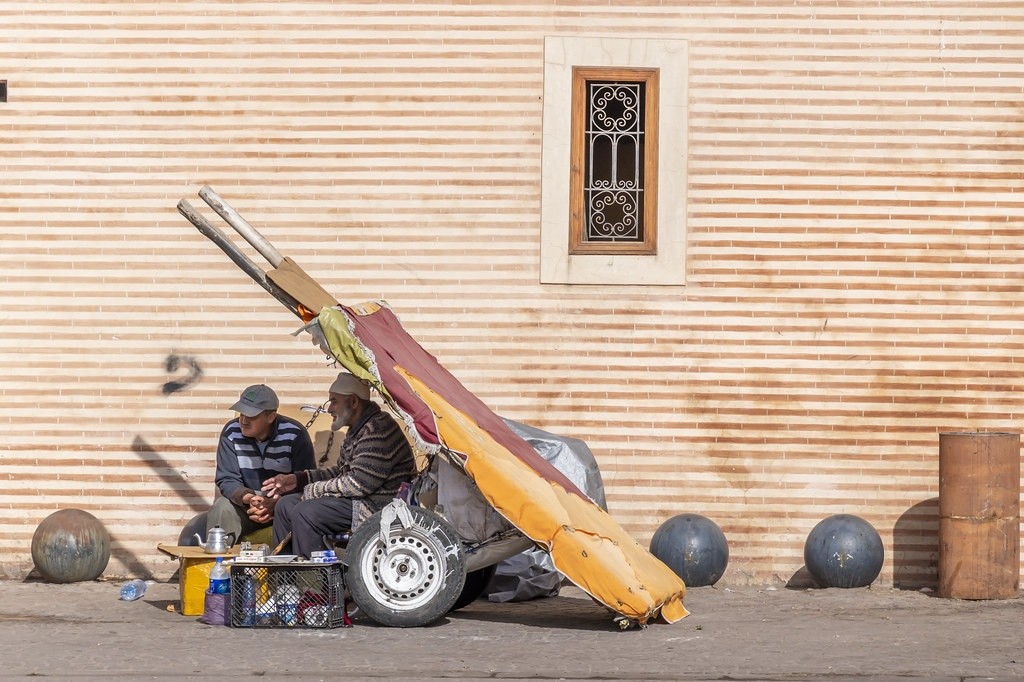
[261,372,418,595]
[206,384,317,546]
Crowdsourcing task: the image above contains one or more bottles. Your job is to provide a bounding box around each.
[242,574,256,625]
[120,578,146,601]
[210,557,231,593]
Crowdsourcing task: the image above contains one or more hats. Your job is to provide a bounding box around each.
[229,384,279,418]
[329,372,370,400]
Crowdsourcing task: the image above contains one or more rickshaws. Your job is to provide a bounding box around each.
[178,186,692,631]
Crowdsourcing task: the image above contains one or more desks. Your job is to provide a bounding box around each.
[158,543,269,616]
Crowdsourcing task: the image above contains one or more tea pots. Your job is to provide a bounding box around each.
[194,525,237,553]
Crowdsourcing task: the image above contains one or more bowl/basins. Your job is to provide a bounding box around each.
[265,554,298,563]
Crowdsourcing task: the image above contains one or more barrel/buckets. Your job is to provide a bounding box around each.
[938,432,1021,600]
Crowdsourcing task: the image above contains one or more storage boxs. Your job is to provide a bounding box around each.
[230,563,345,628]
